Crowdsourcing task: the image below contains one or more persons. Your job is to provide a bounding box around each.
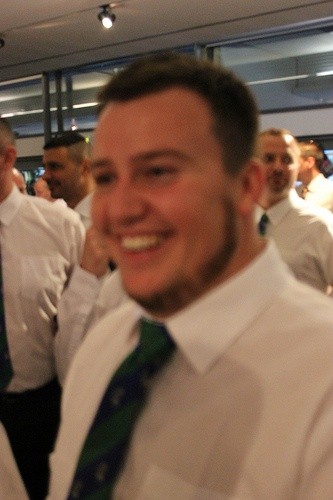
[53,224,130,389]
[311,140,333,178]
[45,52,333,500]
[41,131,117,278]
[251,128,333,298]
[294,140,333,214]
[34,178,68,208]
[11,167,27,195]
[0,117,86,500]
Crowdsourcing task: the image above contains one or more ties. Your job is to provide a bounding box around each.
[67,318,176,500]
[258,215,268,236]
[0,255,15,392]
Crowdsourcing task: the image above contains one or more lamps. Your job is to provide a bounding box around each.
[98,4,116,28]
